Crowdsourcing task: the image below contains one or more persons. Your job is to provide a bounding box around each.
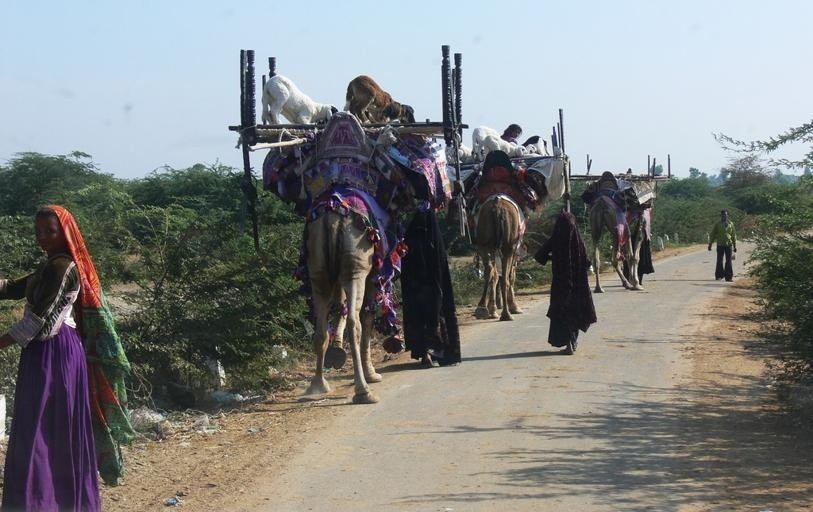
[533,211,597,355]
[499,124,522,145]
[707,210,737,281]
[400,210,461,367]
[0,206,133,509]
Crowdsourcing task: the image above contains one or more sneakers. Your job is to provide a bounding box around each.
[560,346,573,355]
[422,352,433,369]
[572,343,577,351]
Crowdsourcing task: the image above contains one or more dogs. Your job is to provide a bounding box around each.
[343,75,415,125]
[261,74,339,124]
[458,126,551,163]
